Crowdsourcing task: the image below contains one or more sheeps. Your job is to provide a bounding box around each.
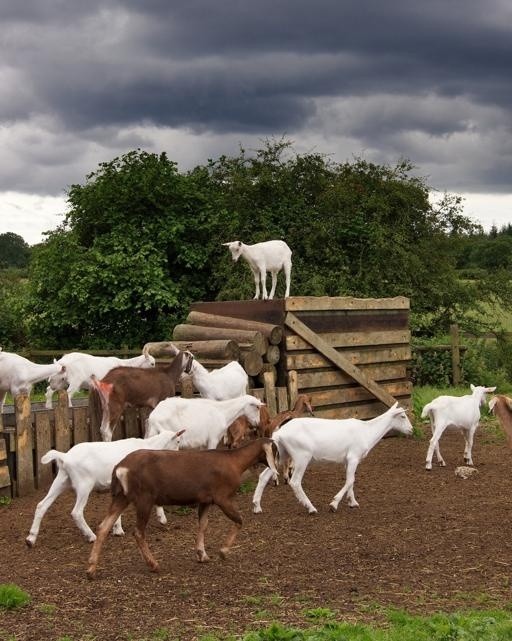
[251,400,415,516]
[221,240,293,301]
[145,395,266,449]
[487,394,512,452]
[90,349,193,442]
[420,383,497,472]
[24,429,187,550]
[265,395,314,468]
[45,345,155,409]
[171,344,249,399]
[86,436,281,581]
[1,350,70,402]
